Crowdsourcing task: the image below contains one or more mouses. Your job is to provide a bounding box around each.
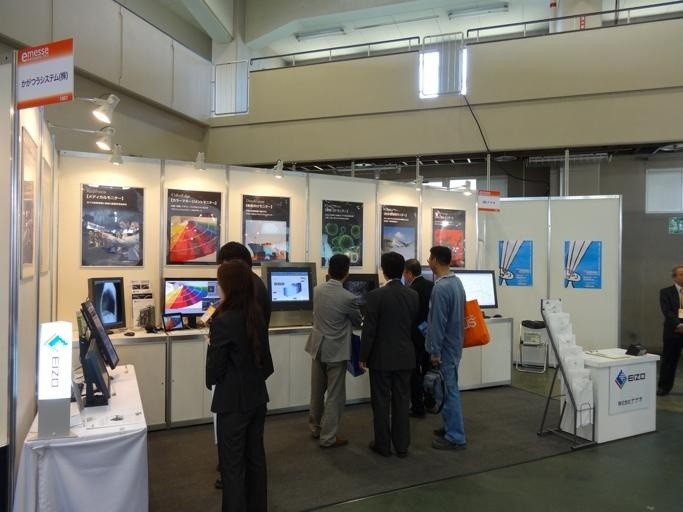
[124,332,134,336]
[494,314,501,317]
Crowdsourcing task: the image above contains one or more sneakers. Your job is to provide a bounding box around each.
[408,408,426,419]
[434,430,445,436]
[432,438,467,450]
[215,478,224,488]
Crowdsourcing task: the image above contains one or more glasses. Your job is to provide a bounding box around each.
[427,256,436,263]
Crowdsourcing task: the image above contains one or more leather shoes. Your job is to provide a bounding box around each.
[397,449,408,458]
[325,436,348,448]
[657,389,668,395]
[369,440,391,457]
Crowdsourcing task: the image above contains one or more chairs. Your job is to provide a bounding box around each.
[515,320,550,374]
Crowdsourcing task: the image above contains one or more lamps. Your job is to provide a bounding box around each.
[273,160,283,180]
[75,93,120,125]
[194,152,206,171]
[47,120,115,150]
[449,4,510,20]
[415,175,424,191]
[109,145,124,166]
[295,28,346,41]
[463,181,472,197]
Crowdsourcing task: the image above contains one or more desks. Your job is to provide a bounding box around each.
[13,364,150,511]
[561,346,660,443]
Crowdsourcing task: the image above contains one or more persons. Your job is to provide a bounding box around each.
[359,251,420,458]
[204,260,270,510]
[403,259,435,419]
[304,254,362,448]
[214,242,274,489]
[85,216,140,266]
[657,267,683,395]
[424,245,467,451]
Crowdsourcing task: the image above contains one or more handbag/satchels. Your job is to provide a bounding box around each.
[345,333,366,377]
[464,299,490,347]
[423,363,448,415]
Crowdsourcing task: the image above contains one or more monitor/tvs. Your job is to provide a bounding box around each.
[401,266,437,286]
[83,339,110,407]
[79,300,119,383]
[261,261,317,327]
[326,274,379,330]
[88,277,126,334]
[449,270,498,318]
[162,278,222,329]
